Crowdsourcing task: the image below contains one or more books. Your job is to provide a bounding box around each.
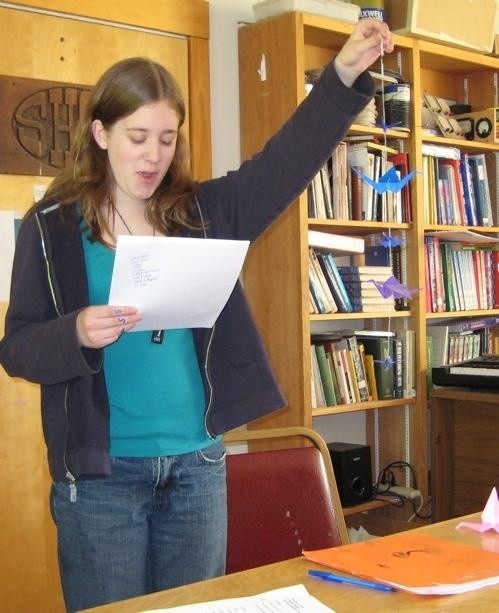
[424,233,498,311]
[423,142,494,227]
[308,227,407,314]
[427,316,498,395]
[309,327,416,408]
[313,132,411,223]
[299,529,499,597]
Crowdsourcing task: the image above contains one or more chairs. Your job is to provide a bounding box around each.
[223,428,351,574]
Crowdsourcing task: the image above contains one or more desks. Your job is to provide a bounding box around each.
[71,511,499,612]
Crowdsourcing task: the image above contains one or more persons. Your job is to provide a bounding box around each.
[0,18,398,610]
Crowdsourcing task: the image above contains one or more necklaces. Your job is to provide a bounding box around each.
[107,200,165,348]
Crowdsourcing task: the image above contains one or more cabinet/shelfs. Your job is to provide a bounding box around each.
[237,16,498,531]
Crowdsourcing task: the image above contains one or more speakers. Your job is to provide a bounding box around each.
[326,441,373,508]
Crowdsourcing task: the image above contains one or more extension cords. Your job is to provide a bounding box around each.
[374,482,422,500]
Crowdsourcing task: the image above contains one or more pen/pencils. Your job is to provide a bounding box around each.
[307,570,400,592]
